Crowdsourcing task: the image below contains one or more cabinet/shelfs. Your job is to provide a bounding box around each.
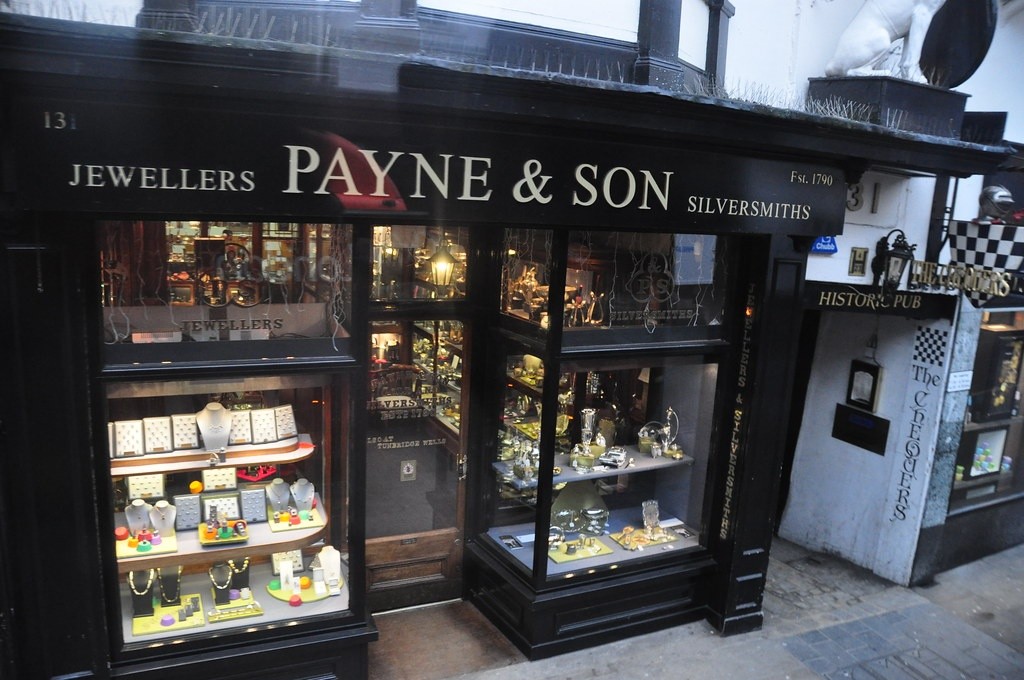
[81,214,379,680]
[373,219,756,663]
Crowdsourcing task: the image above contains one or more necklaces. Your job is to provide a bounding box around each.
[208,424,223,433]
[138,516,141,519]
[129,568,154,596]
[156,507,167,518]
[228,557,249,573]
[292,482,314,503]
[157,566,181,602]
[270,483,284,504]
[209,565,232,589]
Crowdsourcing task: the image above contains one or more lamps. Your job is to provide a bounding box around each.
[870,229,920,301]
[425,225,460,293]
